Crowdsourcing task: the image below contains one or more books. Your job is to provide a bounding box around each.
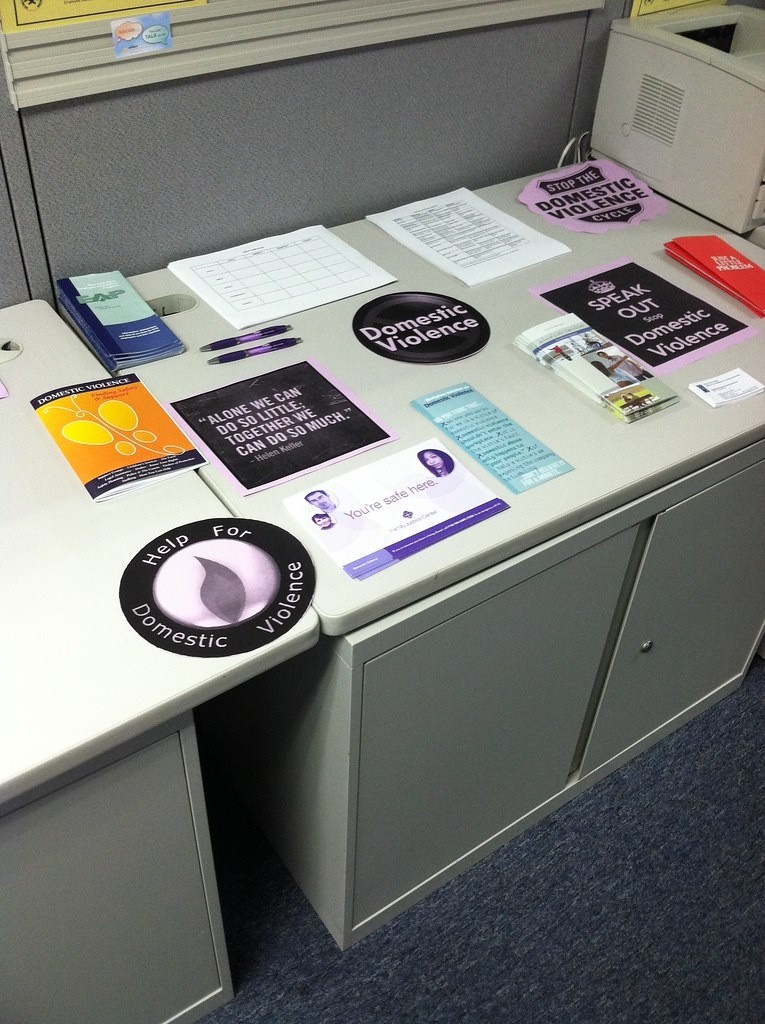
[56,270,184,372]
[30,373,210,502]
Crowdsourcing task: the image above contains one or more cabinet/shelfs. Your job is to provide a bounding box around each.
[1,164,765,1024]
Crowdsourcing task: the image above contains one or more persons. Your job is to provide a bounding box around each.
[571,343,582,353]
[421,449,452,477]
[554,345,573,361]
[583,335,599,349]
[305,490,336,530]
[621,393,647,409]
[597,352,654,381]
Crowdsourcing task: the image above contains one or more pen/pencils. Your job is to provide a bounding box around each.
[199,324,293,352]
[207,337,303,365]
